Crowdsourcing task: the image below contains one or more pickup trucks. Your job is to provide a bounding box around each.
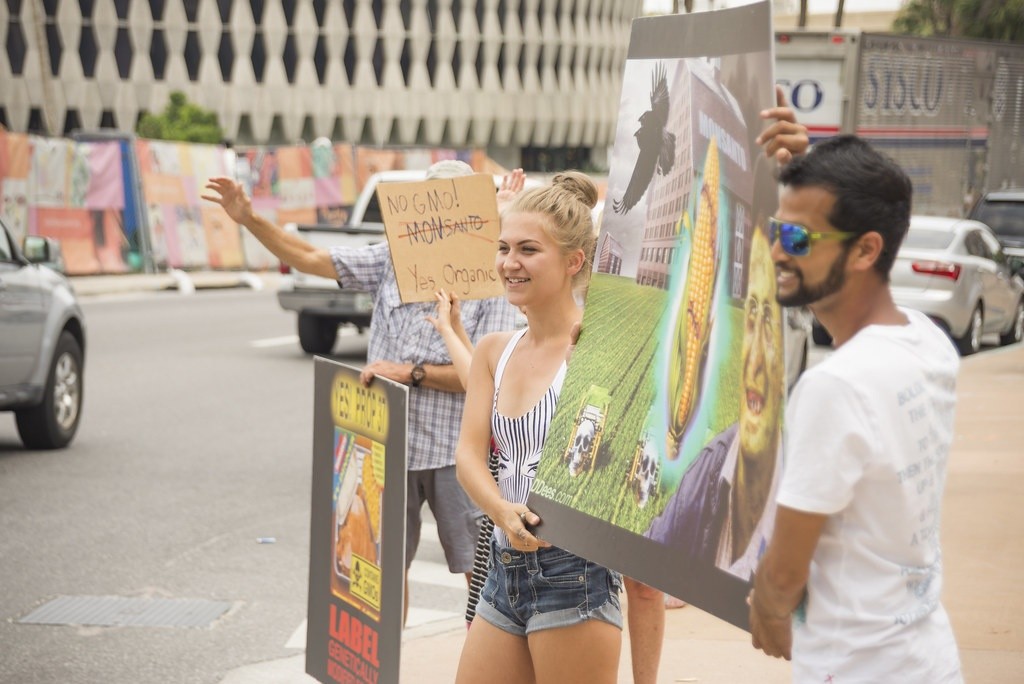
[277,163,549,359]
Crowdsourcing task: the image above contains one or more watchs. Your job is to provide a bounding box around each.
[410,360,426,388]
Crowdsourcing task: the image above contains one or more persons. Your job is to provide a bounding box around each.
[745,85,967,684]
[646,197,807,589]
[198,159,668,684]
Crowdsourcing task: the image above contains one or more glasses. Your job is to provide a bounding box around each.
[768,217,856,256]
[745,315,774,342]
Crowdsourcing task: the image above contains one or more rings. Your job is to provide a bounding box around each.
[521,511,530,520]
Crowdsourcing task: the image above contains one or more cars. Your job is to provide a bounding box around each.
[781,302,815,392]
[961,188,1024,278]
[0,213,91,449]
[810,214,1023,355]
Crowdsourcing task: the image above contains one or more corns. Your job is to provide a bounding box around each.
[664,136,720,459]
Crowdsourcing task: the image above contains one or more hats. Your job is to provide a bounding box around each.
[423,160,473,180]
